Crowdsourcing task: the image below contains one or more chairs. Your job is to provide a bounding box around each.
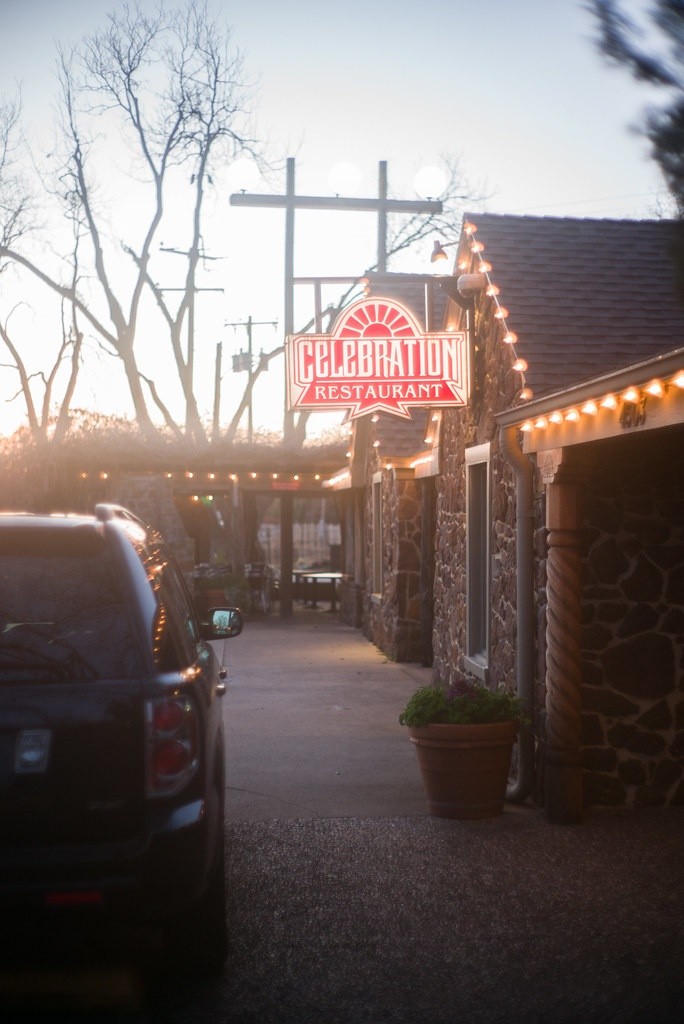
[183,564,276,614]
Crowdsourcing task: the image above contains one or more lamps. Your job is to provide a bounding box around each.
[431,240,460,262]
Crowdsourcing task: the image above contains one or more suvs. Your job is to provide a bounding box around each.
[0,499,246,1024]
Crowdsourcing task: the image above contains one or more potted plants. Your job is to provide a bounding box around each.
[398,686,535,819]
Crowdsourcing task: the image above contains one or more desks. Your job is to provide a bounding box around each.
[305,572,343,615]
[293,569,312,603]
